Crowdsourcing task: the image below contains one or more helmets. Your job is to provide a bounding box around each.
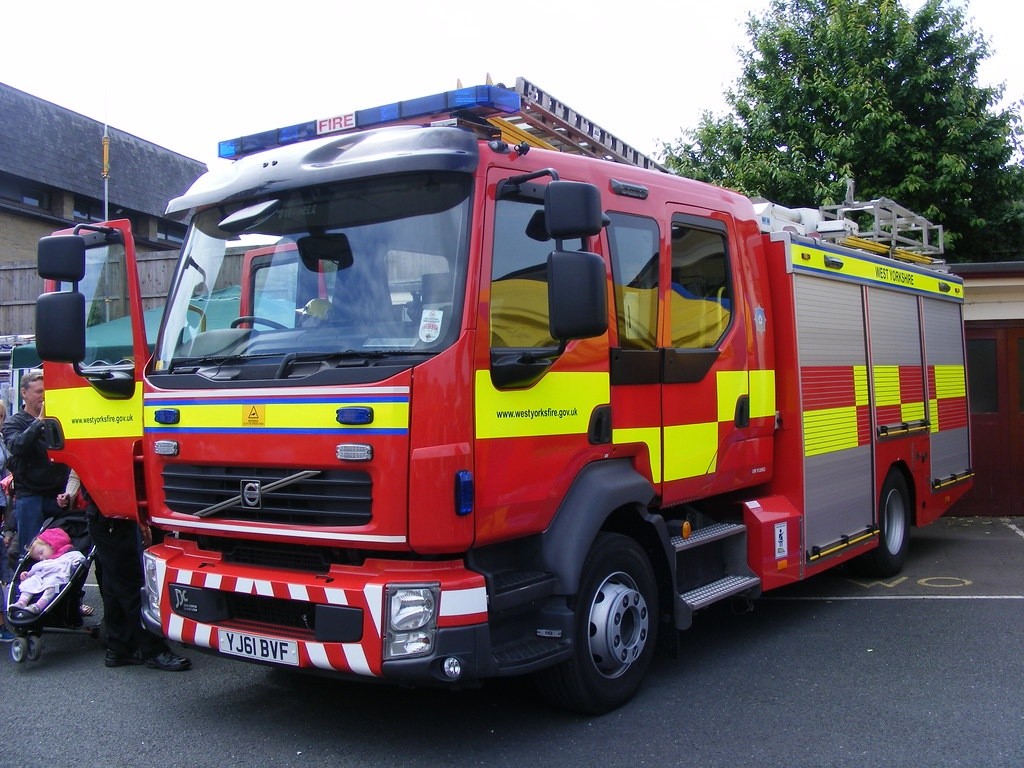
[294,297,334,322]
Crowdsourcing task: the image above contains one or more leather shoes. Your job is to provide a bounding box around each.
[144,650,190,670]
[105,645,145,667]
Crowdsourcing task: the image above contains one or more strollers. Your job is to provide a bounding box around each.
[7,492,98,664]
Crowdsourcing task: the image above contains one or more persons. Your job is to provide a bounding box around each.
[8,528,87,618]
[1,370,94,642]
[57,468,195,673]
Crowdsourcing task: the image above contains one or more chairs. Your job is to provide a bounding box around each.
[330,250,395,324]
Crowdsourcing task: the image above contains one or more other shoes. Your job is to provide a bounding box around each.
[0,625,17,642]
[9,601,27,612]
[24,604,41,614]
[80,604,94,615]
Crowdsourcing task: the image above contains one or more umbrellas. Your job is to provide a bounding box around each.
[10,285,296,369]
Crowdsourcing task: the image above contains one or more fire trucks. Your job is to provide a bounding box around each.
[33,71,974,717]
